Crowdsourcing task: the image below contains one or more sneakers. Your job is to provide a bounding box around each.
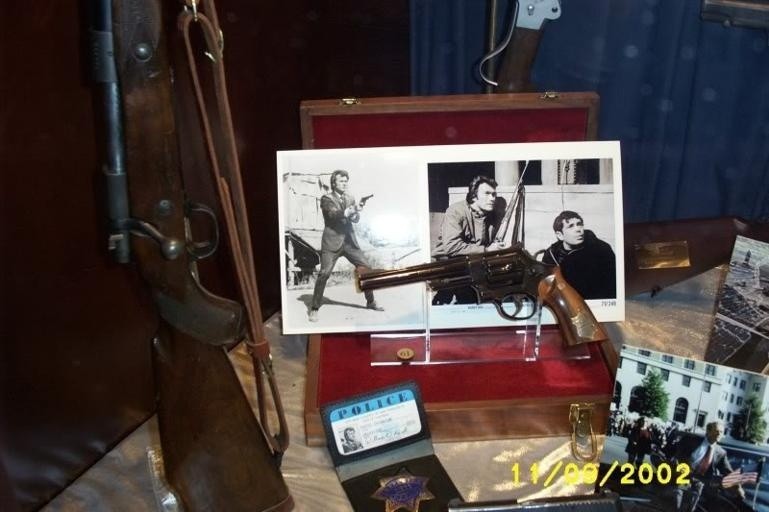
[366,301,384,312]
[308,308,319,323]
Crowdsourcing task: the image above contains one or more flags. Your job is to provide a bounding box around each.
[721,459,764,489]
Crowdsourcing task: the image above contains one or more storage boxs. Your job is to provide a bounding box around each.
[299,88,618,448]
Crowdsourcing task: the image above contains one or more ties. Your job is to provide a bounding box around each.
[697,445,713,477]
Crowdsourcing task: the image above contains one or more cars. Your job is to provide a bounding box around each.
[652,433,768,511]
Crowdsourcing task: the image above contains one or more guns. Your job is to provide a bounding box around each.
[359,242,608,347]
[493,161,529,242]
[360,195,372,206]
[93,0,296,511]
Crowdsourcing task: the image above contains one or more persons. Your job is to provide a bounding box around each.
[308,170,386,322]
[608,402,747,512]
[542,211,615,299]
[342,427,363,455]
[430,174,506,307]
